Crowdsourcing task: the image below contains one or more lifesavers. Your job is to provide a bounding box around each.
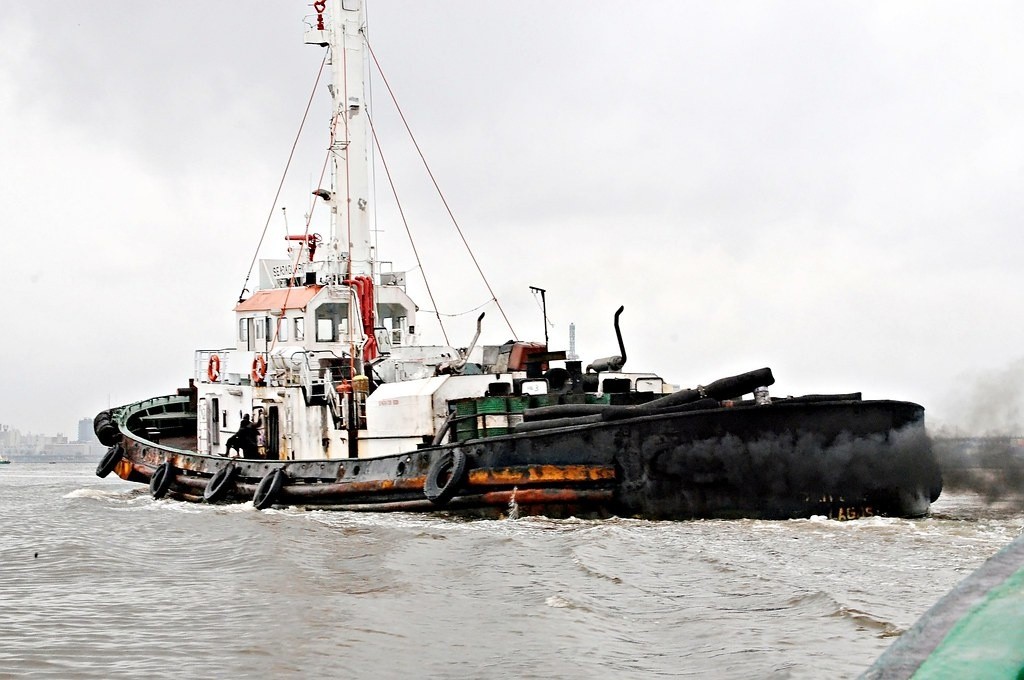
[95,442,124,478]
[421,448,467,503]
[251,467,285,510]
[148,461,174,498]
[208,354,220,381]
[203,463,236,504]
[251,355,266,385]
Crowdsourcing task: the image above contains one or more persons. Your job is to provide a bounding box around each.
[217,414,262,460]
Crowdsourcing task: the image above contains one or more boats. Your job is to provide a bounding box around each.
[0,459,11,464]
[93,0,945,521]
[49,461,56,464]
